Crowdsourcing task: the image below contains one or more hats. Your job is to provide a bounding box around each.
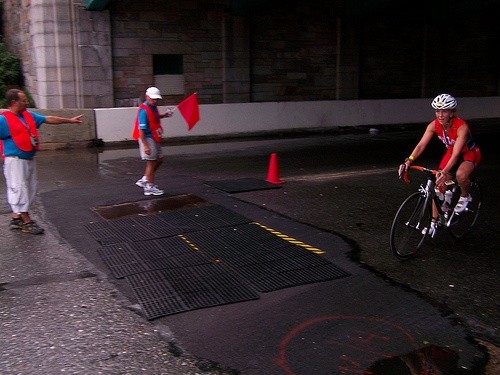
[146,86,163,100]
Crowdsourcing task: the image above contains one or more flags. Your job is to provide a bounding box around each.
[177,94,200,131]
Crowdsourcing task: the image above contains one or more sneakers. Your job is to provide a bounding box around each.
[10,217,23,227]
[136,176,150,186]
[454,194,473,213]
[421,223,439,237]
[141,185,165,197]
[18,220,44,235]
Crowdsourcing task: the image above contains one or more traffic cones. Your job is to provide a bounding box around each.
[264,153,285,185]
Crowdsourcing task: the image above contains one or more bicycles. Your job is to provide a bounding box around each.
[389,164,483,262]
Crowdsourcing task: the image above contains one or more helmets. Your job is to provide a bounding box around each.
[432,93,458,110]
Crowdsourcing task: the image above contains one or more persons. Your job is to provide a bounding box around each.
[133,87,174,195]
[398,94,481,234]
[0,89,85,234]
